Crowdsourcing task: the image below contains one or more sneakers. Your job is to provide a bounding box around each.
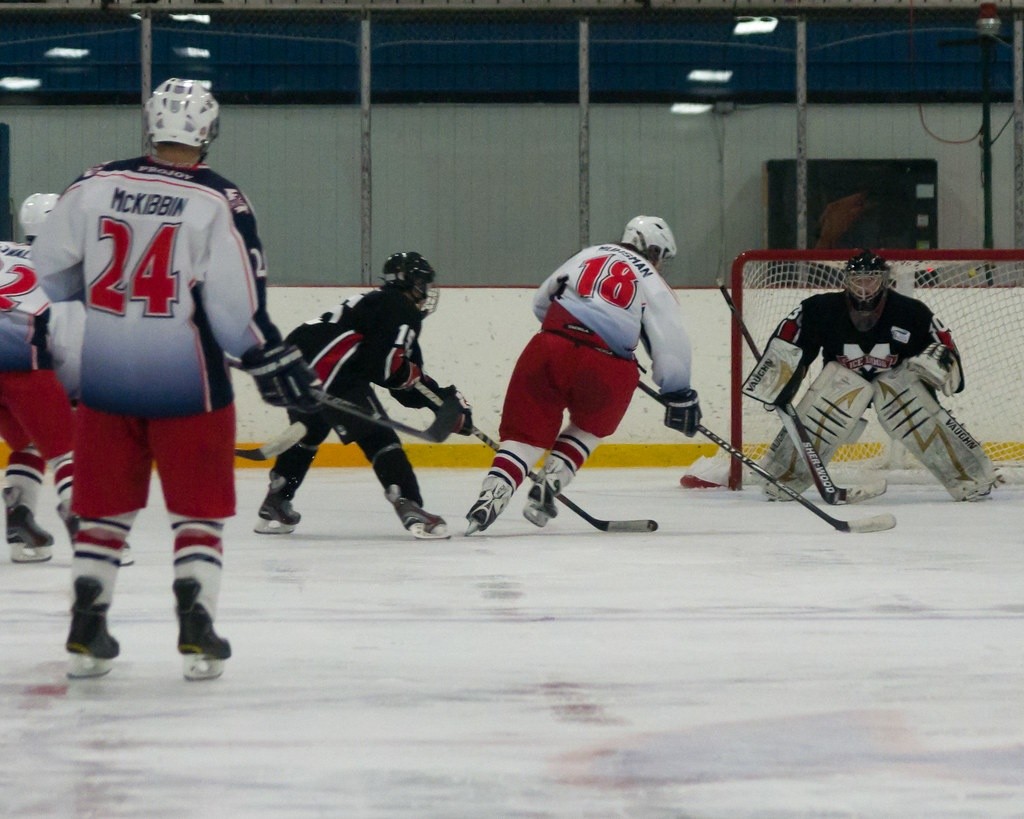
[382,481,453,539]
[52,575,119,681]
[56,497,138,565]
[251,470,302,537]
[165,575,231,680]
[1,485,56,564]
[461,475,514,536]
[523,455,570,527]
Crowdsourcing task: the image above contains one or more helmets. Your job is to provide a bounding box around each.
[16,190,62,242]
[621,214,678,266]
[846,249,893,308]
[377,252,440,317]
[145,75,221,151]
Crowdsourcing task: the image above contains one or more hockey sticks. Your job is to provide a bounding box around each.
[637,381,898,535]
[227,358,464,444]
[412,380,661,536]
[715,276,889,506]
[233,420,308,463]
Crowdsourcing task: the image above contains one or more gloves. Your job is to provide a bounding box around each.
[243,338,330,418]
[664,388,703,438]
[386,371,442,409]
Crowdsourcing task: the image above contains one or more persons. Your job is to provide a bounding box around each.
[0,192,136,566]
[29,77,322,681]
[466,215,701,537]
[743,256,1003,503]
[252,252,472,540]
